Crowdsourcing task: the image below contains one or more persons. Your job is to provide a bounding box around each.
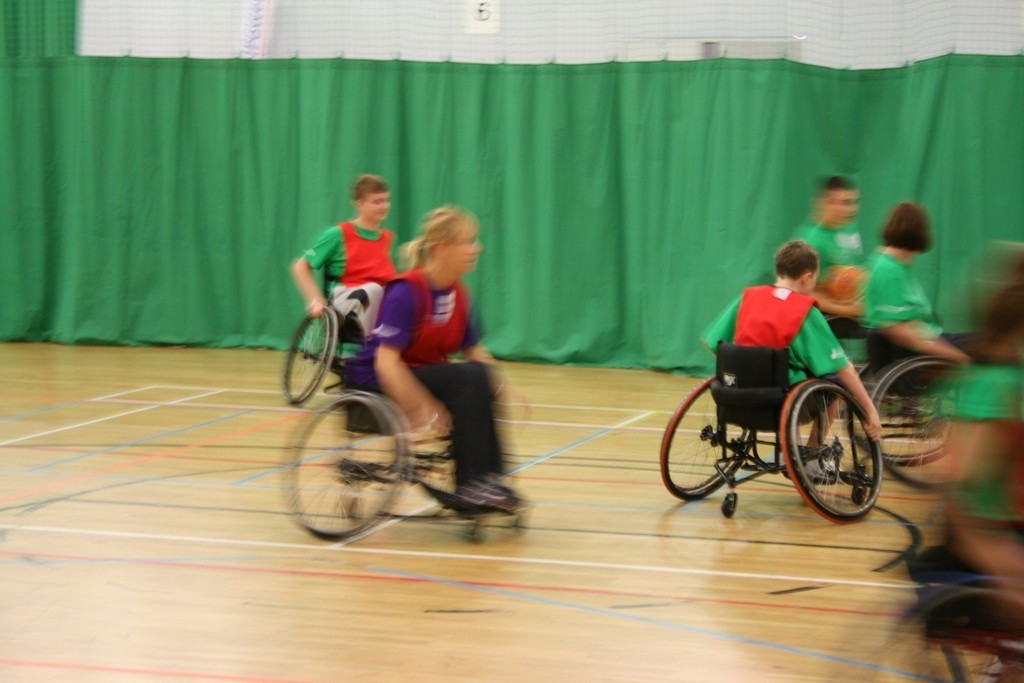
[861,204,980,392]
[290,172,400,344]
[343,209,522,516]
[701,239,885,483]
[789,175,871,341]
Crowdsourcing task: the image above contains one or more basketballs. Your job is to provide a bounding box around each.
[825,266,865,303]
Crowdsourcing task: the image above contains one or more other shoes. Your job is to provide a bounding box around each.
[456,477,516,511]
[805,459,836,481]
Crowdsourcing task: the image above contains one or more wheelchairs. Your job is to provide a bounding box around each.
[660,340,883,524]
[827,317,966,492]
[282,263,533,543]
[897,543,1024,683]
